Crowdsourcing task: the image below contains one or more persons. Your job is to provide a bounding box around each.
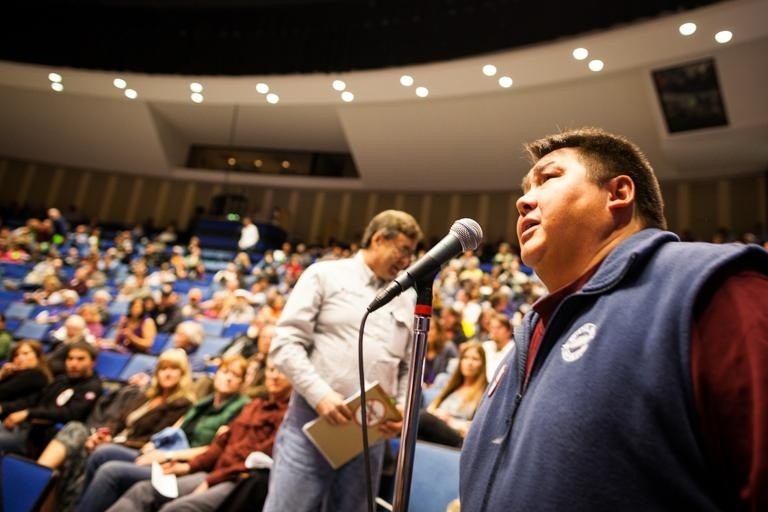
[457,126,768,511]
[674,225,768,252]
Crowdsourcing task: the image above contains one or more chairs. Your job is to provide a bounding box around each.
[0,236,270,511]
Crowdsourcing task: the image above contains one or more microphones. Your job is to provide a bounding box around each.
[368,218,483,312]
